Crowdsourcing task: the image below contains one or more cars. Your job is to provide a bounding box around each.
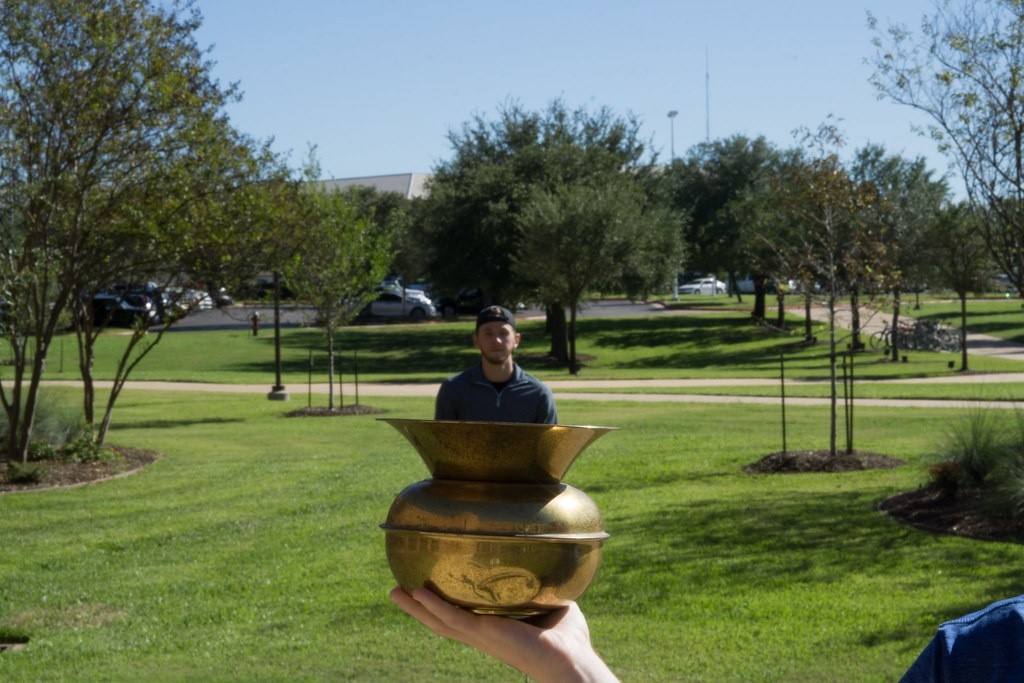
[726,269,777,295]
[57,275,295,331]
[365,276,494,324]
[677,275,728,297]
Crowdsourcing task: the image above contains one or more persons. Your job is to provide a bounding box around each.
[389,586,1024,683]
[217,288,233,309]
[435,306,558,425]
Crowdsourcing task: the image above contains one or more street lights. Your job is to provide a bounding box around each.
[668,110,678,161]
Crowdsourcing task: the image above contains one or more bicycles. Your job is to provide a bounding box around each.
[869,312,963,354]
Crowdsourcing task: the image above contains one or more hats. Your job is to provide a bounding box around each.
[477,306,515,328]
